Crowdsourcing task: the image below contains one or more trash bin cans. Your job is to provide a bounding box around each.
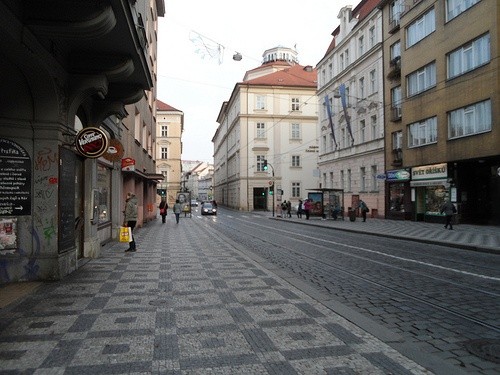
[349,209,356,222]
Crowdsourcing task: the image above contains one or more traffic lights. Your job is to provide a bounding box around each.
[264,160,268,171]
[262,192,265,199]
[269,186,273,194]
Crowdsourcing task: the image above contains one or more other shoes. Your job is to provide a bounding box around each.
[125,247,136,252]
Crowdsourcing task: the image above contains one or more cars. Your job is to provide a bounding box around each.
[191,200,199,206]
[201,203,216,216]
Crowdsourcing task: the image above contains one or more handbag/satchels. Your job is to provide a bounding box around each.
[119,225,133,243]
[160,209,164,213]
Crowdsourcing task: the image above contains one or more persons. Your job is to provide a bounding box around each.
[280,199,369,223]
[178,194,187,210]
[122,192,182,253]
[440,199,457,230]
